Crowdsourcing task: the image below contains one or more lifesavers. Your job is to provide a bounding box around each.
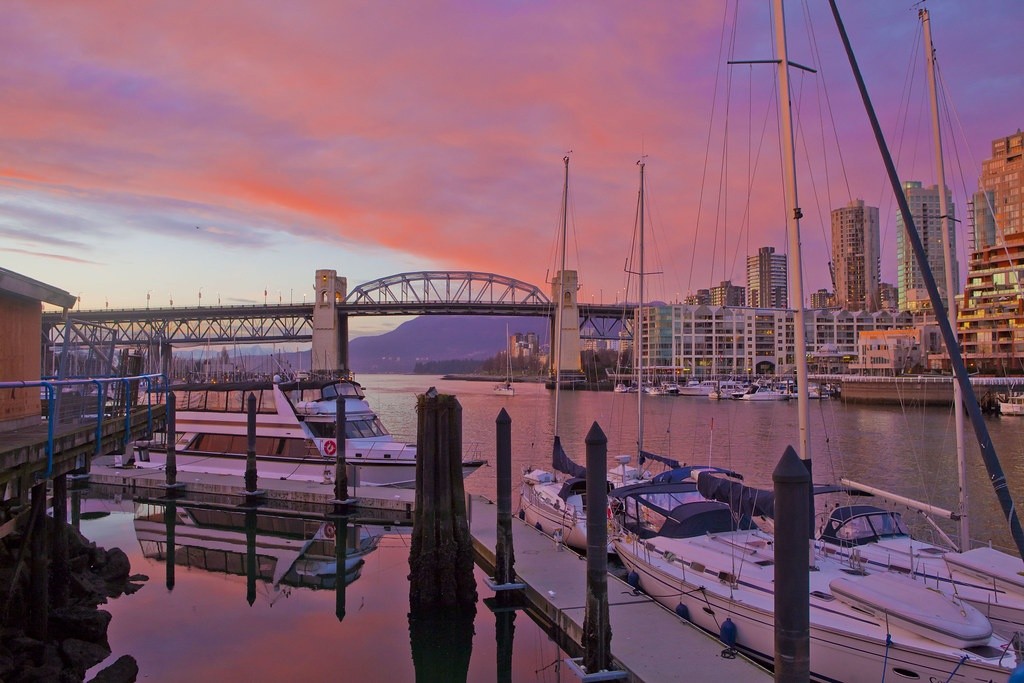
[324,523,335,539]
[324,440,336,455]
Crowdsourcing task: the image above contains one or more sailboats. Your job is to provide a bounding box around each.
[519,149,728,555]
[751,0,1024,643]
[492,323,514,396]
[607,0,1024,683]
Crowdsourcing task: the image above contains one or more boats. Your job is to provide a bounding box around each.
[40,379,202,409]
[1000,392,1024,416]
[133,355,487,490]
[614,380,835,401]
[132,503,414,609]
